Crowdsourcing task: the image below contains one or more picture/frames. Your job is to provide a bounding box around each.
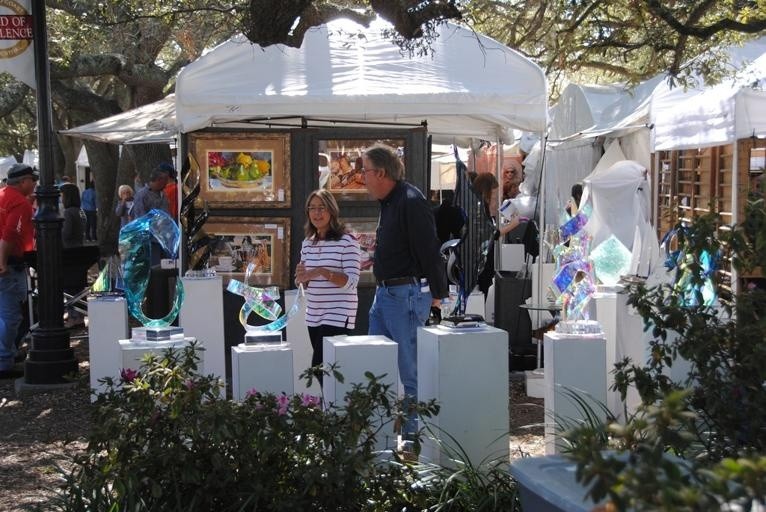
[188,215,291,295]
[310,132,409,204]
[186,131,291,209]
[339,216,385,287]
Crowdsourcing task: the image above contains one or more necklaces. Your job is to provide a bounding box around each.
[315,236,328,255]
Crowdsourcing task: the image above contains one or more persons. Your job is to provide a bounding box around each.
[565,184,584,249]
[434,186,468,289]
[133,169,168,219]
[115,185,134,231]
[161,162,178,225]
[324,156,362,189]
[82,179,96,241]
[361,144,449,463]
[58,183,87,328]
[470,172,521,302]
[294,188,361,391]
[502,161,523,201]
[0,163,40,381]
[32,171,65,247]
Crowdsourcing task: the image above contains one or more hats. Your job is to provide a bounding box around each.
[2,163,39,184]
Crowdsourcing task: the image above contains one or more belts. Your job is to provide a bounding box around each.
[375,277,413,286]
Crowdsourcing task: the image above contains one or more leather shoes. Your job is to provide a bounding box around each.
[14,349,26,361]
[1,367,24,380]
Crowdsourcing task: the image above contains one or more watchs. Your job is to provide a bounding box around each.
[329,269,331,284]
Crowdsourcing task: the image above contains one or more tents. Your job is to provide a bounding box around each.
[519,82,651,251]
[651,37,766,324]
[58,93,175,146]
[174,7,546,371]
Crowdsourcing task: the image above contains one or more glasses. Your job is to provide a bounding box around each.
[361,167,380,175]
[307,206,327,212]
